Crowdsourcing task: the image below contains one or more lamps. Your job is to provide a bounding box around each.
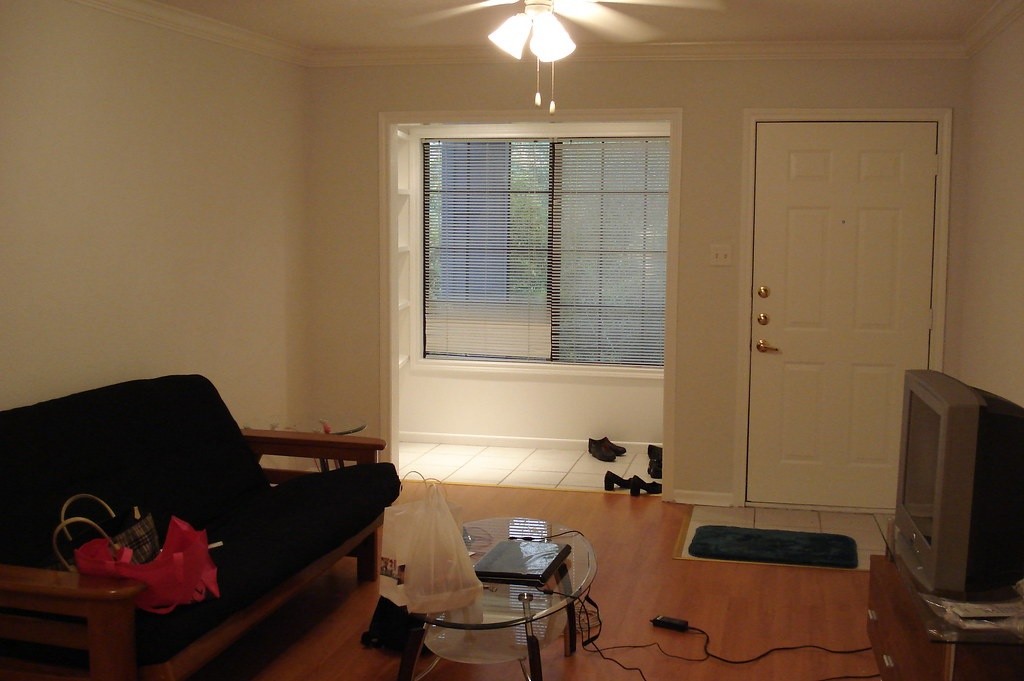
[489,1,578,117]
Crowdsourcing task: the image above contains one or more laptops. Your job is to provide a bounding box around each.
[474,540,572,587]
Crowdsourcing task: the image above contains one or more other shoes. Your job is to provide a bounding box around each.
[589,437,627,456]
[647,445,663,479]
[591,442,617,461]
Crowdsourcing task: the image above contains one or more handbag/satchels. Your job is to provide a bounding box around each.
[51,494,160,575]
[378,469,481,615]
[74,516,221,615]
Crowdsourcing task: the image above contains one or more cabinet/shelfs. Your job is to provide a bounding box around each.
[866,555,1024,681]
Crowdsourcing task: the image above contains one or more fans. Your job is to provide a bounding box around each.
[403,0,727,44]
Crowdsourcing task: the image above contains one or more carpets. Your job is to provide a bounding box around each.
[687,525,859,570]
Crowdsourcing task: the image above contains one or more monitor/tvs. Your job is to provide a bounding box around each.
[893,369,1024,595]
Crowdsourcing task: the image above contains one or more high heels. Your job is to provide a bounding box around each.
[604,469,634,491]
[629,474,662,496]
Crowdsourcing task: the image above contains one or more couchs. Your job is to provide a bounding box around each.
[0,373,404,681]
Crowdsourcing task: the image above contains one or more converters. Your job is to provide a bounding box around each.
[652,616,688,632]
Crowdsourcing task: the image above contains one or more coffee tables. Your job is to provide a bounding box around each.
[393,517,596,680]
